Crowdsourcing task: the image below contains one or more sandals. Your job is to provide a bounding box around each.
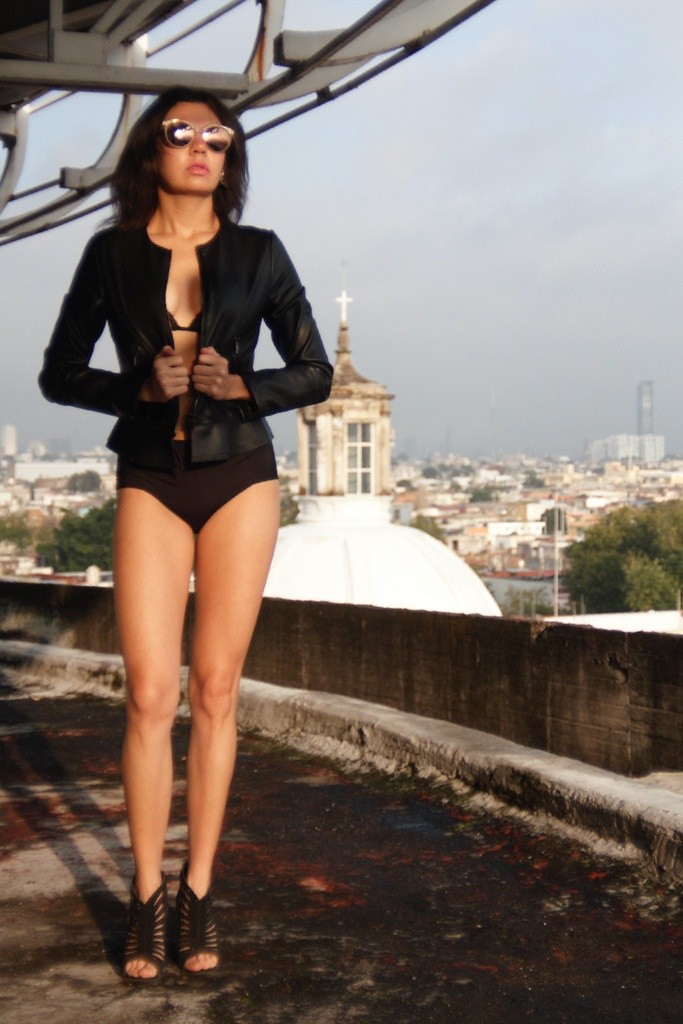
[174,862,221,976]
[123,871,168,983]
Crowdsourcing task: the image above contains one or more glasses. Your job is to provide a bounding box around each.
[161,118,236,154]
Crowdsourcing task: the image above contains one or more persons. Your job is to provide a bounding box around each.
[40,88,334,983]
